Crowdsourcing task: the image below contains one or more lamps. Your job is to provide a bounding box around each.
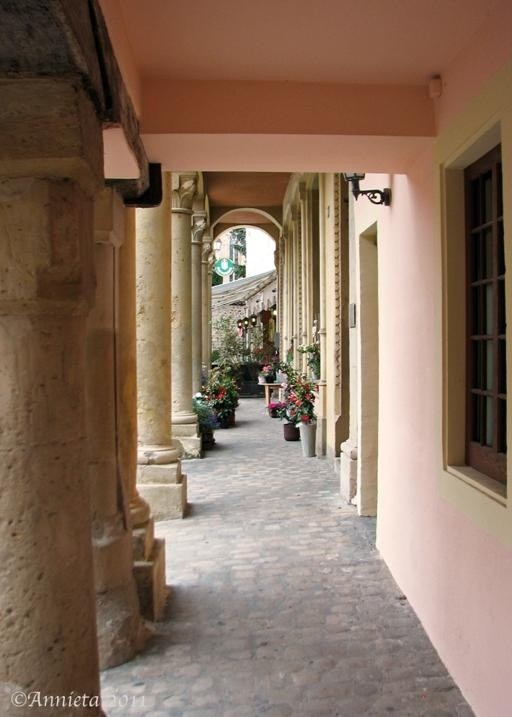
[237,312,257,329]
[343,172,391,205]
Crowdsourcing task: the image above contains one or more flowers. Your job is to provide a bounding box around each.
[267,402,287,408]
[258,361,284,377]
[193,370,240,430]
[276,340,320,423]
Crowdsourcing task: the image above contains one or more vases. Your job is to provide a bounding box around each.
[201,429,215,451]
[266,377,274,383]
[283,424,316,457]
[269,409,277,418]
